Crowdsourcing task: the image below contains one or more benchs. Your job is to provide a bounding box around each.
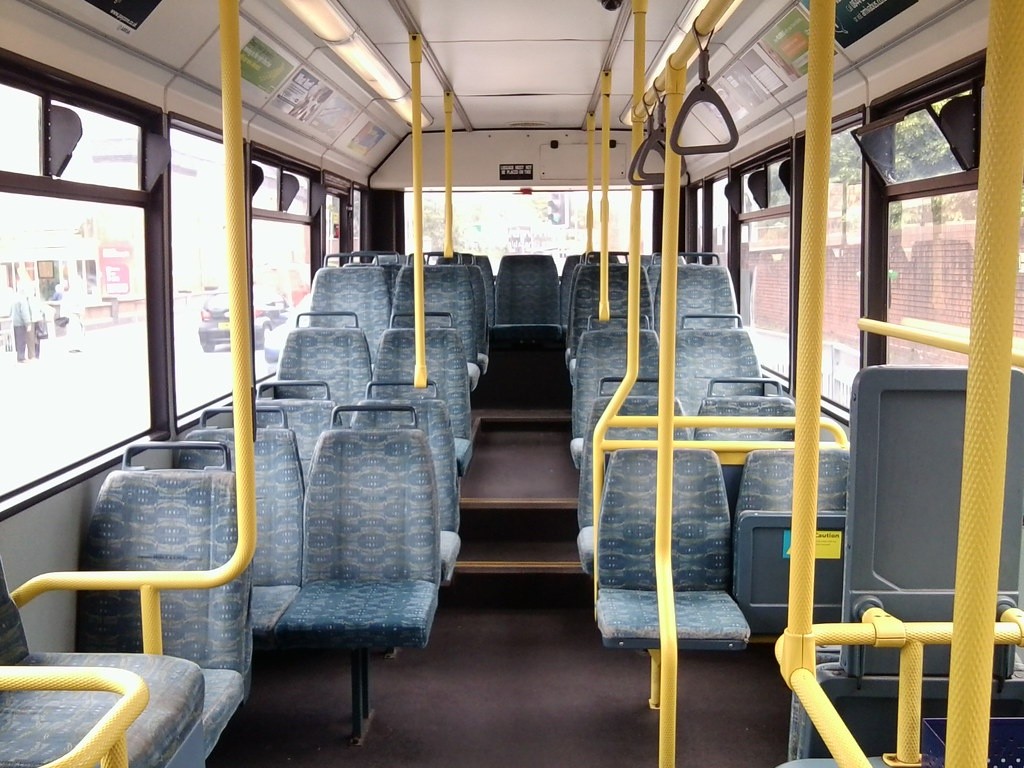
[175,252,853,746]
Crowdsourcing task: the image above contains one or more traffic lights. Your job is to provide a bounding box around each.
[547,192,569,228]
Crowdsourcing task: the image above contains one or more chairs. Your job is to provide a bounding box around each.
[788,365,1024,762]
[1,556,206,768]
[76,442,253,759]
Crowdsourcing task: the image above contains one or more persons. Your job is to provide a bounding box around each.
[11,281,45,365]
[50,273,84,354]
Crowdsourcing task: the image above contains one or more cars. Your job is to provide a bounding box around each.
[199,291,291,352]
[254,265,313,372]
